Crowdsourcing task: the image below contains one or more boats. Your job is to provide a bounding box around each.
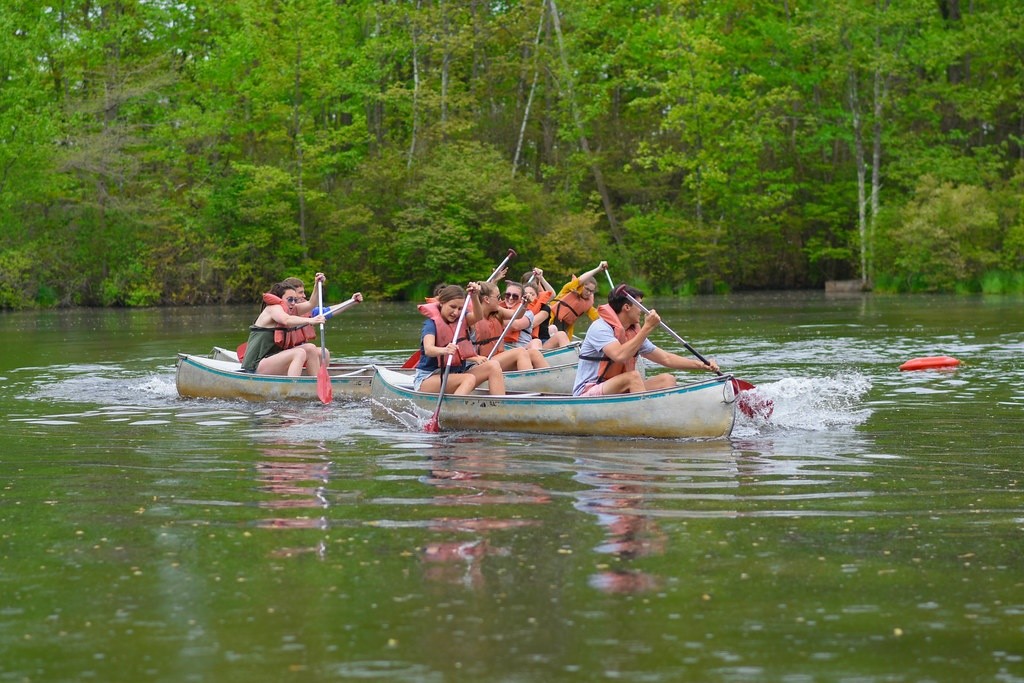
[175,357,646,404]
[370,366,737,439]
[212,341,583,366]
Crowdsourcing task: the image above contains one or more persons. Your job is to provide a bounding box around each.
[469,267,570,370]
[414,282,506,396]
[572,285,719,396]
[239,273,327,378]
[284,278,363,367]
[550,260,608,342]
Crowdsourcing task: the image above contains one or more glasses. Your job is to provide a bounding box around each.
[504,293,519,300]
[282,296,298,303]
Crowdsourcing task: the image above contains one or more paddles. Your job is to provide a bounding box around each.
[488,297,527,360]
[615,284,774,420]
[316,273,333,402]
[486,248,517,282]
[425,285,473,432]
[295,296,361,330]
[602,264,615,291]
[526,271,536,282]
